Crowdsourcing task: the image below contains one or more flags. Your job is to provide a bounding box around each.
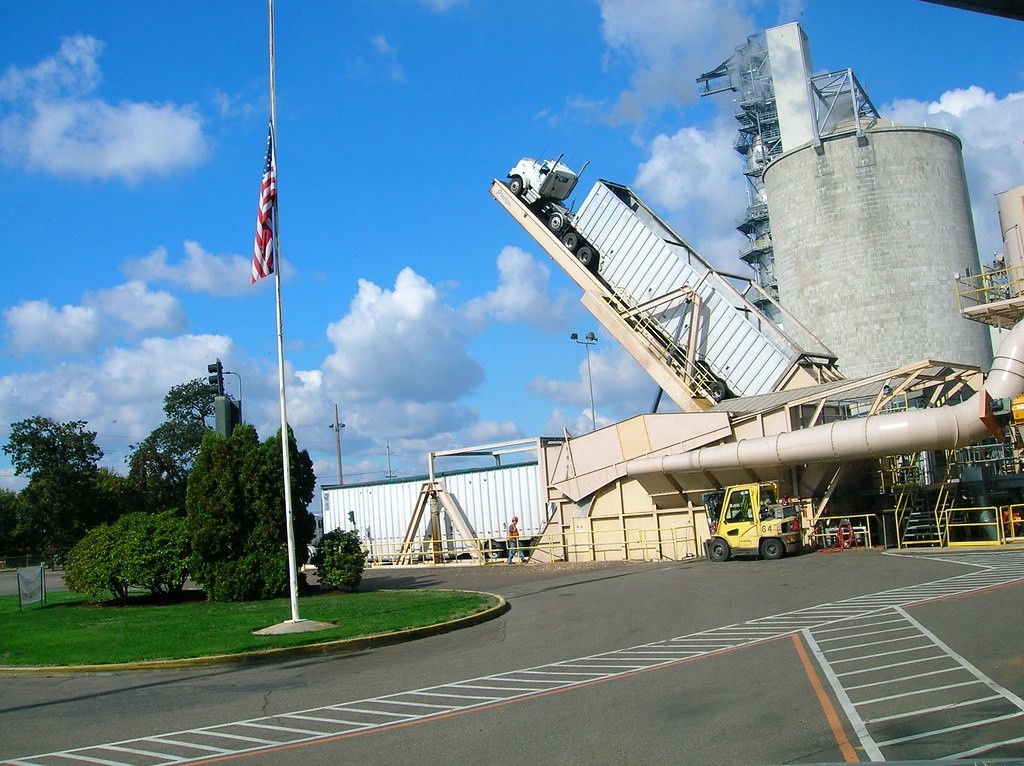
[250,116,279,284]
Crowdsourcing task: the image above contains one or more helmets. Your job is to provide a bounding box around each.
[513,517,518,521]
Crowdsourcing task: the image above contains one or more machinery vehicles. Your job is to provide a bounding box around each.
[702,479,809,562]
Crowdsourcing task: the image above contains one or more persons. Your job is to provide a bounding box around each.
[507,517,528,564]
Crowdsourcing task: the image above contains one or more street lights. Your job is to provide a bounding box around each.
[329,403,346,486]
[572,331,600,430]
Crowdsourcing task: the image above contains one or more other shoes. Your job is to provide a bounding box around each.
[522,559,527,563]
[508,562,516,564]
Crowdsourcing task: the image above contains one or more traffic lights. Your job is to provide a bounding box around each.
[208,358,224,395]
